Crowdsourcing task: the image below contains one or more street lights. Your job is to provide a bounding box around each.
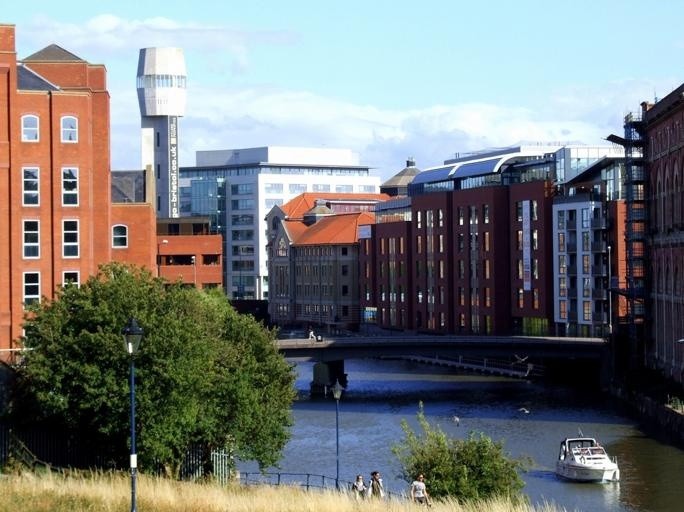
[606,245,613,334]
[329,377,349,494]
[156,238,168,277]
[191,256,197,287]
[119,312,148,512]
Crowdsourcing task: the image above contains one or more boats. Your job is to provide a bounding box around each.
[553,435,624,485]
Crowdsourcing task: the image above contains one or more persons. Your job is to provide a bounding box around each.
[367,470,384,501]
[352,475,367,499]
[410,472,431,508]
[307,325,316,340]
